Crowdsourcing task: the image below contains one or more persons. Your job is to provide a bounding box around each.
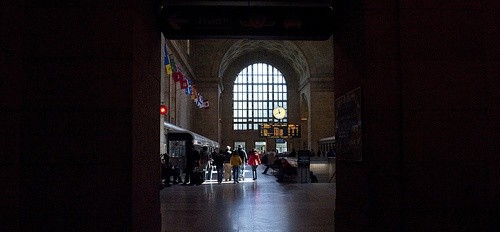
[274,157,294,183]
[213,149,226,184]
[248,148,261,180]
[326,149,335,163]
[181,144,248,187]
[308,148,321,161]
[229,150,242,184]
[161,154,184,189]
[261,147,296,175]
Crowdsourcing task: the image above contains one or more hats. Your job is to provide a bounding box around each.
[226,146,231,149]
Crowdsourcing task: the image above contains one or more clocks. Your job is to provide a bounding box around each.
[272,107,286,119]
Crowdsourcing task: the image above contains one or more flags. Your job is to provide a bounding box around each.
[163,43,210,112]
